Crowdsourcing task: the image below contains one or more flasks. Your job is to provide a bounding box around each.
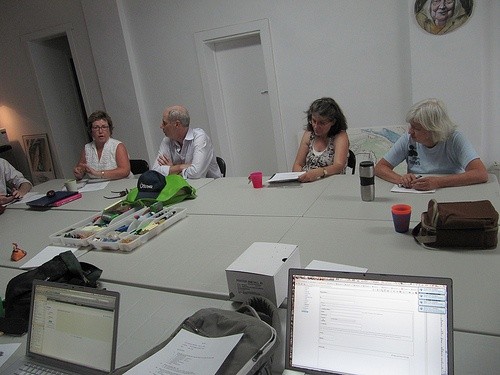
[360,161,375,201]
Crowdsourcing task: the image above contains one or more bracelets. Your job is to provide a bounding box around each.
[101,170,104,178]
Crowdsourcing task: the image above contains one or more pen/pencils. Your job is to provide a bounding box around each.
[134,223,148,235]
[164,211,176,220]
[401,174,422,187]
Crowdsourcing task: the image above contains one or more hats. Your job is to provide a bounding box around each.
[135,169,167,200]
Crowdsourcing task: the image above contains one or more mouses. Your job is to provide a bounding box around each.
[47,189,55,198]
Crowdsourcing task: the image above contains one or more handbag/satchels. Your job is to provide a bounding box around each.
[2,249,104,335]
[412,199,499,248]
[109,295,279,375]
[128,173,197,206]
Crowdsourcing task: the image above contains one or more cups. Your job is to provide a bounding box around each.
[248,171,263,188]
[64,179,77,192]
[391,204,412,233]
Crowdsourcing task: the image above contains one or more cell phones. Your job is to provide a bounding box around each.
[5,194,12,197]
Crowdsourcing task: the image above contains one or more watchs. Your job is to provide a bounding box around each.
[322,167,327,178]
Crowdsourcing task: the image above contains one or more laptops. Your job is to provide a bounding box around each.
[281,267,454,375]
[9,279,120,375]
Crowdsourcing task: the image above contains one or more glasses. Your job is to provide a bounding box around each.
[91,124,111,131]
[309,118,332,126]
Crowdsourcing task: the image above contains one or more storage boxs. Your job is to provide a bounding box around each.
[224,242,301,309]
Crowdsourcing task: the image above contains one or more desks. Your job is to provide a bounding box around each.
[0,172,500,375]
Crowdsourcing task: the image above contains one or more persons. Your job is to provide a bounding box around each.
[0,158,33,205]
[152,105,223,179]
[292,97,349,183]
[375,98,488,191]
[74,111,135,180]
